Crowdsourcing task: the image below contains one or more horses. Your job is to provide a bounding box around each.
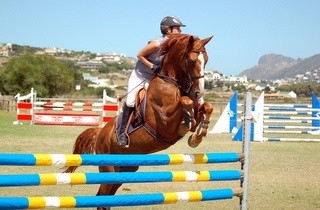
[63,31,213,210]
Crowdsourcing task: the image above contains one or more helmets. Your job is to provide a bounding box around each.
[160,16,188,31]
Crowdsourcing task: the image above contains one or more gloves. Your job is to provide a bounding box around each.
[150,65,163,74]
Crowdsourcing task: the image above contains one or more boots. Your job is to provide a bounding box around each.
[117,102,135,146]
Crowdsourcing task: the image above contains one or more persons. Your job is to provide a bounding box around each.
[118,16,186,147]
